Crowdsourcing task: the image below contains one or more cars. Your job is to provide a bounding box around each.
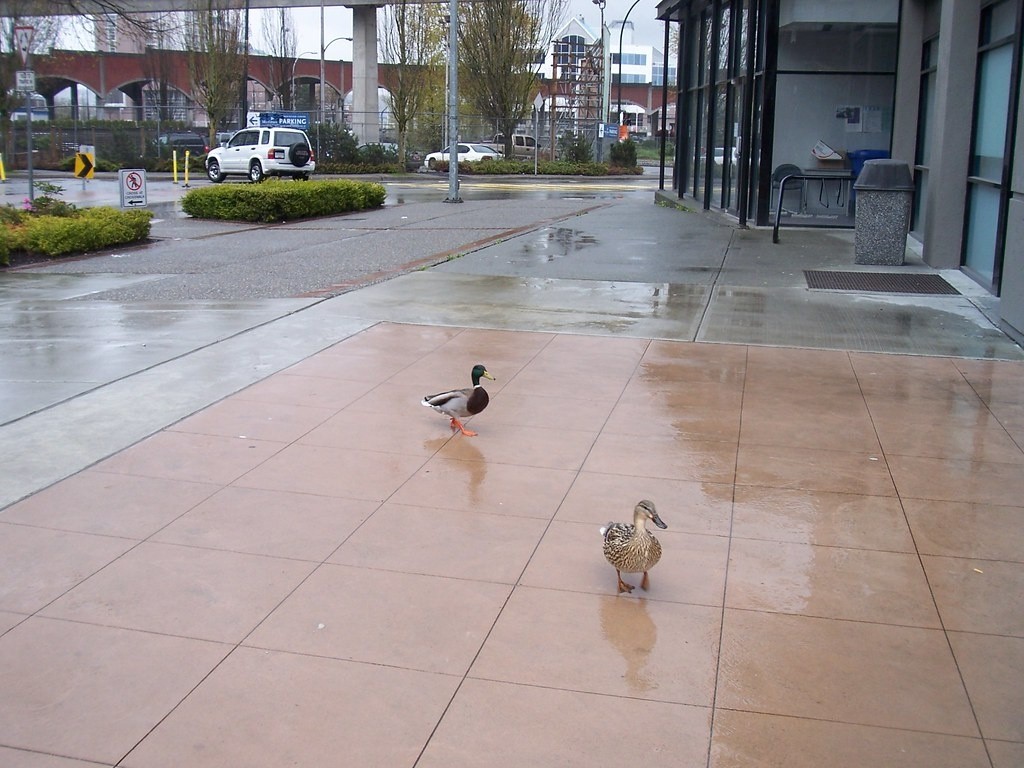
[424,142,506,171]
[696,146,761,168]
[152,130,240,156]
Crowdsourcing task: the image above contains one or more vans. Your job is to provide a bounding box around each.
[492,133,545,163]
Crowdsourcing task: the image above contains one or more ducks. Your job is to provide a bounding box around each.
[599,499,668,595]
[420,365,496,438]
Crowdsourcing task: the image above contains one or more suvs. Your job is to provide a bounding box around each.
[205,124,316,185]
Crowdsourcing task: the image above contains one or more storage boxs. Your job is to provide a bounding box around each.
[815,148,849,169]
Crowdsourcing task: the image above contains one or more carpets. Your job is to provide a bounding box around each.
[802,269,963,296]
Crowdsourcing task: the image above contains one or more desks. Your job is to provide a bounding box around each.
[801,168,852,216]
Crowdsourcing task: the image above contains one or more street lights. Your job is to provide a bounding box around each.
[320,37,354,146]
[593,0,607,123]
[292,50,318,113]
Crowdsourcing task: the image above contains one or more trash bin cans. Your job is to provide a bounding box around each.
[848,149,891,189]
[853,158,915,265]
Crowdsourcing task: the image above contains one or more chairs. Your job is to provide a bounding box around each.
[769,164,804,214]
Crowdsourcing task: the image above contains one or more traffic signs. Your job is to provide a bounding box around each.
[247,112,311,129]
